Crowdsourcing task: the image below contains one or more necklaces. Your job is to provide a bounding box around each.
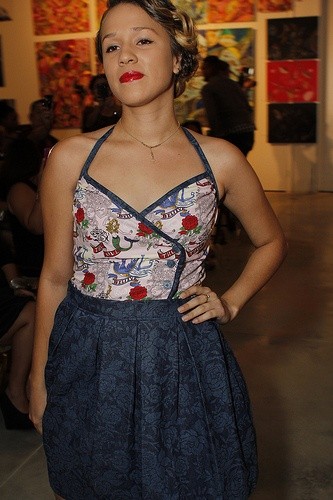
[118,122,180,159]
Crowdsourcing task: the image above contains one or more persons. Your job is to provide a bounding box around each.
[182,55,255,270]
[28,0,292,500]
[80,74,122,133]
[0,96,63,431]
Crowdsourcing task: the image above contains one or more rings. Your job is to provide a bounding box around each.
[203,293,210,303]
